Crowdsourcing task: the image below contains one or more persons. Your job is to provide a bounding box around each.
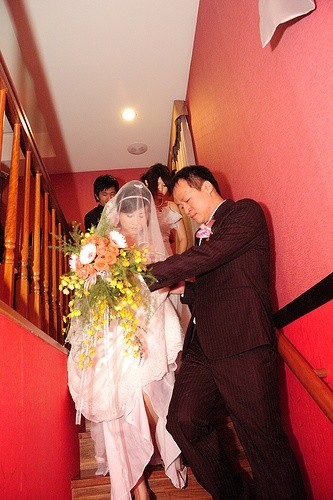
[139,163,187,320]
[137,165,308,500]
[14,228,59,325]
[84,174,120,234]
[68,180,187,500]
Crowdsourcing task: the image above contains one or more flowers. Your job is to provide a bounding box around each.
[47,203,159,371]
[196,224,211,239]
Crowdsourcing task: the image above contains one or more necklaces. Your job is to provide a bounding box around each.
[155,198,163,208]
